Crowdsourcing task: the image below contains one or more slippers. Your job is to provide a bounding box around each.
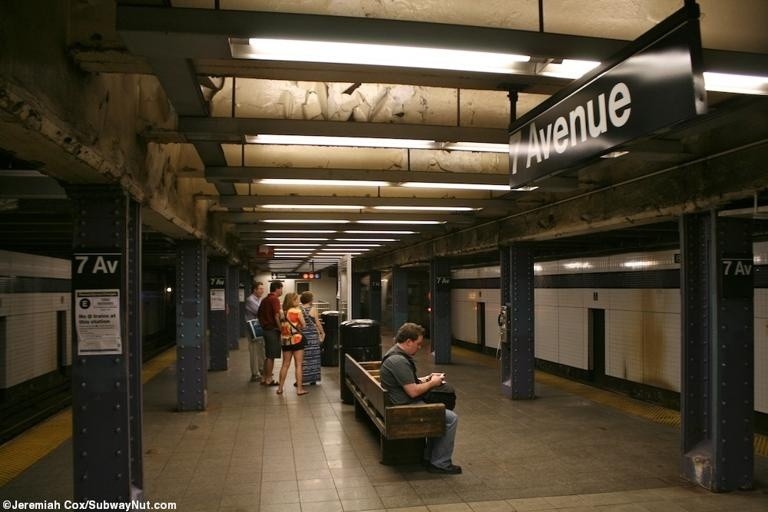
[251,372,316,395]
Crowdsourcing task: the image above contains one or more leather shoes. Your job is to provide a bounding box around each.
[423,459,462,474]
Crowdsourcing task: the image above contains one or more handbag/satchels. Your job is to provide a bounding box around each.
[421,382,456,410]
[318,333,324,343]
[301,335,307,346]
[247,319,264,341]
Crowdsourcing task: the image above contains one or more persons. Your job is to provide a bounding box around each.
[258,282,282,387]
[277,293,309,395]
[244,282,274,382]
[380,322,462,474]
[294,291,325,386]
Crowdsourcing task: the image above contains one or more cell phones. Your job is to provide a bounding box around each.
[440,373,447,377]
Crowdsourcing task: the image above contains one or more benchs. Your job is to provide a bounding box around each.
[345,352,445,466]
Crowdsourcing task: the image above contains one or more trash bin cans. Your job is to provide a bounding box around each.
[321,310,345,366]
[341,319,383,403]
